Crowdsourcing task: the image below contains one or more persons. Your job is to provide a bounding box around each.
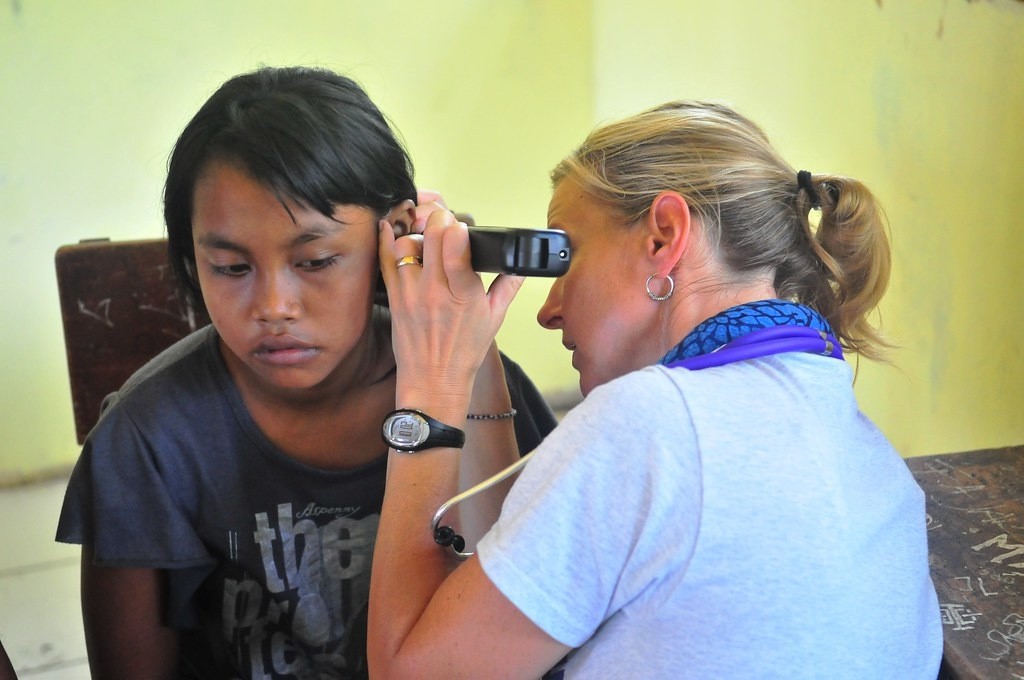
[367,102,944,680]
[56,65,561,680]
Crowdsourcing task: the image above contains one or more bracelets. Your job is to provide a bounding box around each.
[467,408,517,420]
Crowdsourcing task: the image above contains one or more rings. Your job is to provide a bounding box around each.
[395,255,423,269]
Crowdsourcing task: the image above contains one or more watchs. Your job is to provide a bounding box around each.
[380,408,465,454]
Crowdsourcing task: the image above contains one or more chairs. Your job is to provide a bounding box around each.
[54,209,475,446]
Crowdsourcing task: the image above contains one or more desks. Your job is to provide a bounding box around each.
[902,444,1024,680]
